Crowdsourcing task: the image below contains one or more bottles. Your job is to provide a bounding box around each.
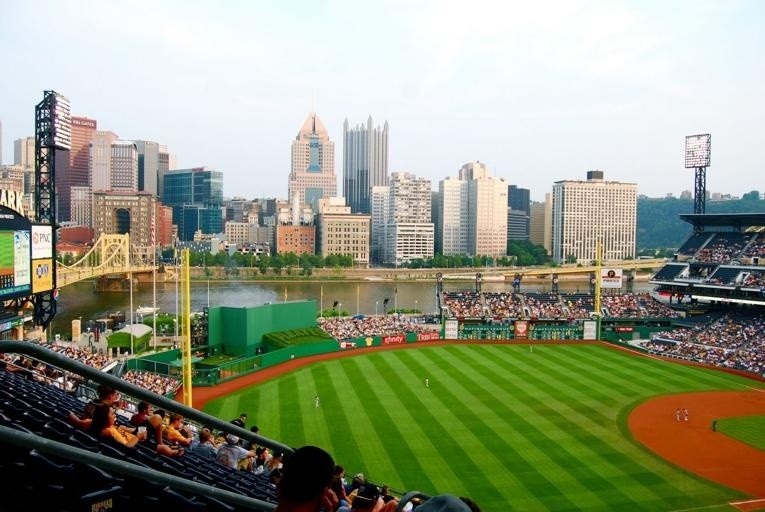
[337,500,350,512]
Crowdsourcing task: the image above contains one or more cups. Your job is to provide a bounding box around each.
[138,426,146,441]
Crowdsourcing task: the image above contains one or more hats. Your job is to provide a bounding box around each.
[226,432,240,444]
[255,446,267,457]
[413,496,470,511]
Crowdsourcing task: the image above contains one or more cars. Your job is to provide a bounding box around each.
[147,260,171,268]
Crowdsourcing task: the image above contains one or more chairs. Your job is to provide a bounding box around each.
[0,370,278,512]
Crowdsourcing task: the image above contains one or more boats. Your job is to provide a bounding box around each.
[190,311,208,320]
[136,305,161,316]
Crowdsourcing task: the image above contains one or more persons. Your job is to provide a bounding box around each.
[425,378,429,387]
[318,313,442,341]
[439,289,679,326]
[677,235,763,292]
[683,408,688,421]
[120,369,181,396]
[70,384,283,488]
[713,420,718,432]
[3,339,111,402]
[314,396,320,408]
[638,310,763,377]
[277,445,481,510]
[530,343,533,353]
[676,408,680,422]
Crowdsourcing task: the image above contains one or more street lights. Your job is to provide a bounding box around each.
[338,303,342,320]
[685,133,711,234]
[33,90,72,329]
[414,300,418,314]
[375,301,379,317]
